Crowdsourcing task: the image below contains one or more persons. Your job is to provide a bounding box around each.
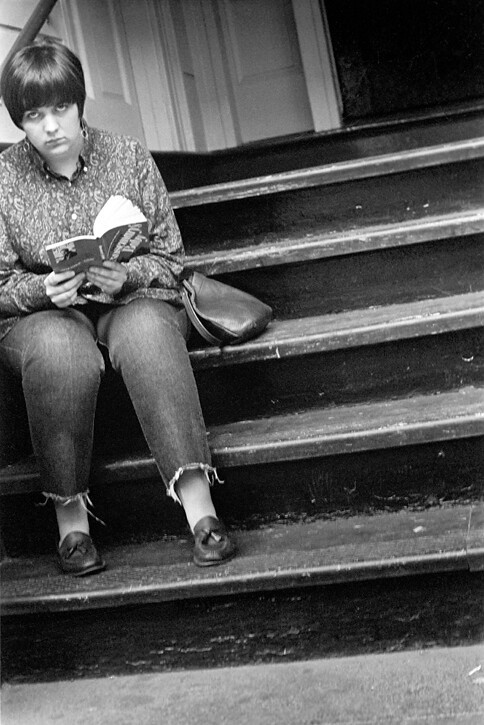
[0,38,240,577]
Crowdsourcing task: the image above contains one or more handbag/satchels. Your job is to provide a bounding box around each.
[176,265,273,346]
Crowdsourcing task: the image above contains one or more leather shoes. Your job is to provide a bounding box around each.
[188,516,237,566]
[54,532,106,577]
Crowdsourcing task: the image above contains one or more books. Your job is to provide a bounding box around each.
[44,195,150,285]
